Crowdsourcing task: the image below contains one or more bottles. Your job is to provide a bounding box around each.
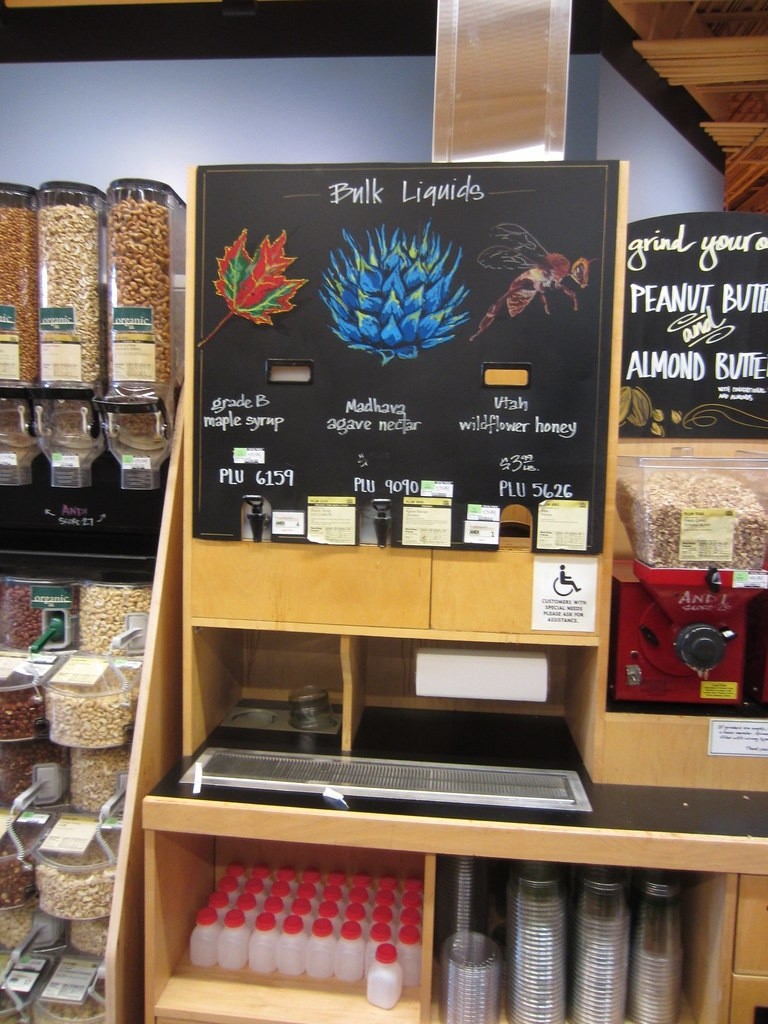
[363,943,405,1008]
[189,862,422,985]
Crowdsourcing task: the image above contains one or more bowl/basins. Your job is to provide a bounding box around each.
[435,852,685,1024]
[287,684,335,732]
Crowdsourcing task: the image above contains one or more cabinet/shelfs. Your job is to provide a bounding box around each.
[143,731,764,1019]
